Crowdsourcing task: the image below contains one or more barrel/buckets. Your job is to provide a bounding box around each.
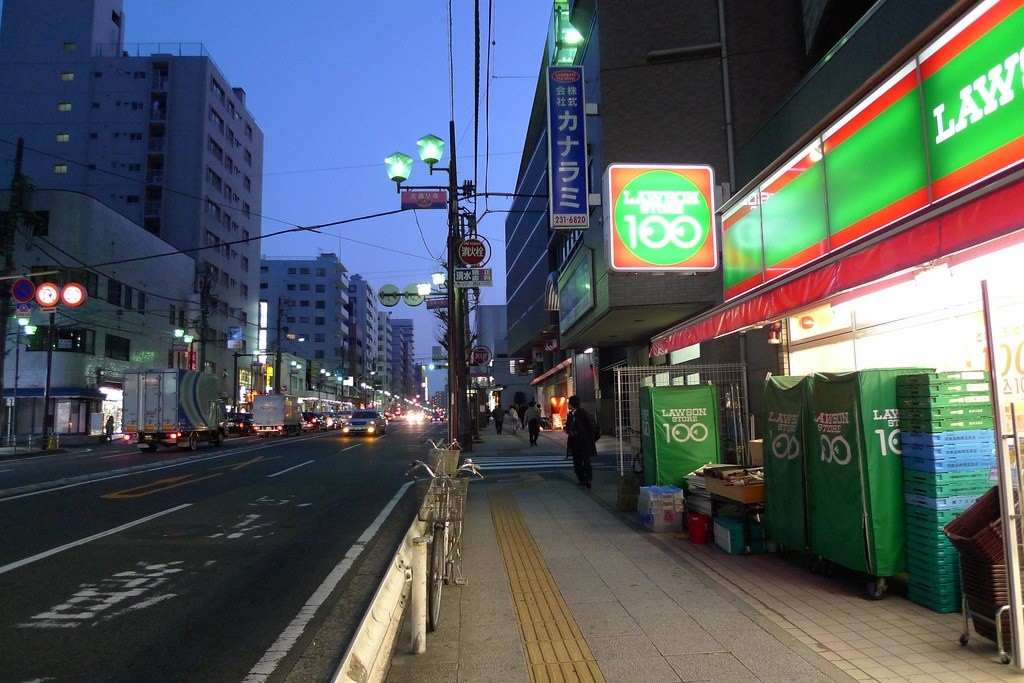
[688,513,709,544]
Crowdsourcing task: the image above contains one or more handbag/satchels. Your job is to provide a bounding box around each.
[513,410,518,420]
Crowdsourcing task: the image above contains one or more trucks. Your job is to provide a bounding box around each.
[253,395,302,438]
[121,368,228,453]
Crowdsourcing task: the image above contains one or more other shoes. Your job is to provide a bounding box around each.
[582,479,592,489]
[531,444,533,446]
[533,440,537,445]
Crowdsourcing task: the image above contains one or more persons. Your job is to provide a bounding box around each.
[105,416,114,442]
[563,395,601,488]
[486,405,491,425]
[509,404,517,434]
[516,399,542,446]
[491,403,505,435]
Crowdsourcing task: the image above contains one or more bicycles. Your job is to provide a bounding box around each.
[401,438,484,631]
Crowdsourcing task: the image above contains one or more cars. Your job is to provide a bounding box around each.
[344,408,387,436]
[229,408,444,437]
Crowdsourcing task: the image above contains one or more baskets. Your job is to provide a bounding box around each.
[942,485,1024,649]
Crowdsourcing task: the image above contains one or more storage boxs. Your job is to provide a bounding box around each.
[750,438,764,467]
[896,370,1023,650]
[639,486,683,532]
[703,474,764,503]
[713,516,764,555]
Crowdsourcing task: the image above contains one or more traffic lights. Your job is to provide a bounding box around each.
[429,363,441,370]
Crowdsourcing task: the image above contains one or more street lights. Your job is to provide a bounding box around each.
[354,371,375,405]
[384,0,461,439]
[174,328,184,368]
[288,361,302,394]
[318,369,330,400]
